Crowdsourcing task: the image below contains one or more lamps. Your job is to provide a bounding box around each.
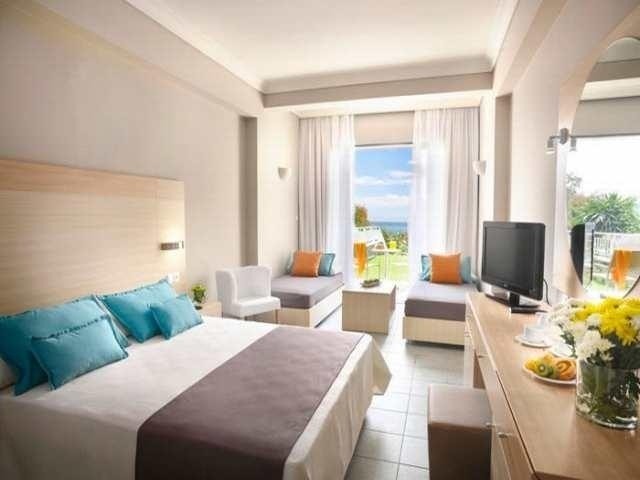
[278,165,292,181]
[544,129,563,157]
[563,128,578,152]
[472,161,487,175]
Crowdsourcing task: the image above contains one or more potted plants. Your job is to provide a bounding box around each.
[191,283,205,306]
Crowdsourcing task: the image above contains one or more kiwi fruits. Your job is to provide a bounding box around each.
[534,360,553,376]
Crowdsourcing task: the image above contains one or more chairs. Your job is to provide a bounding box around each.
[211,262,282,324]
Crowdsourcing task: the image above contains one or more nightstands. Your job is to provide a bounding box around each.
[196,299,224,320]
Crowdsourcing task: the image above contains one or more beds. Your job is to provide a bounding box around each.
[0,157,372,480]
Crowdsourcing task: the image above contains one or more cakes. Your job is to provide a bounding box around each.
[556,359,576,381]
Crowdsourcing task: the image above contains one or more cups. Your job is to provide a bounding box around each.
[523,323,547,342]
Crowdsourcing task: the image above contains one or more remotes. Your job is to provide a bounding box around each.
[510,306,546,312]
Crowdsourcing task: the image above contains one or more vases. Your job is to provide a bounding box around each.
[573,353,638,435]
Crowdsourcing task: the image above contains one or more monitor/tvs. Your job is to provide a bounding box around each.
[480,220,546,307]
[572,222,592,286]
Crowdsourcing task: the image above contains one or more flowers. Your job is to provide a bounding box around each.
[556,291,639,421]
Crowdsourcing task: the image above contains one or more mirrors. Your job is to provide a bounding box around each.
[562,35,639,302]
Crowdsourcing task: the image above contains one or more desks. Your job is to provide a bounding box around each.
[341,280,397,336]
[460,290,638,478]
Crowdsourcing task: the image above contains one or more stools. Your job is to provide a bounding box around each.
[426,380,492,479]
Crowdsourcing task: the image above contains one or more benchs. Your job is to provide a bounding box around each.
[249,271,346,327]
[401,274,479,348]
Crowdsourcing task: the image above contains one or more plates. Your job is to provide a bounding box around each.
[520,358,576,384]
[360,279,382,286]
[514,334,553,348]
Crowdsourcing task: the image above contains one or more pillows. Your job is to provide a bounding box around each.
[318,252,337,278]
[417,253,472,284]
[28,313,129,392]
[1,290,133,396]
[99,276,175,345]
[428,253,461,286]
[288,249,320,278]
[150,293,203,344]
